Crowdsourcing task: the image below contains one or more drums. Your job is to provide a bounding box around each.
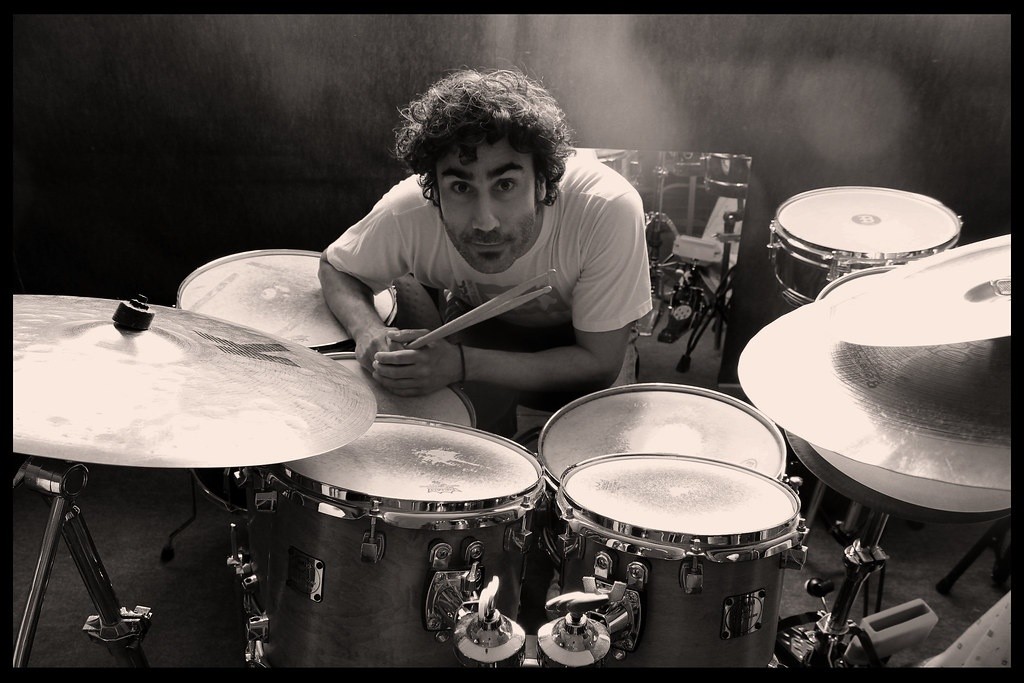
[705,153,749,200]
[176,247,399,514]
[783,265,1011,526]
[772,185,963,306]
[246,414,549,666]
[551,454,809,668]
[536,383,789,568]
[319,352,477,430]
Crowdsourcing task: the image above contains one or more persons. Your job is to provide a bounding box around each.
[319,68,653,635]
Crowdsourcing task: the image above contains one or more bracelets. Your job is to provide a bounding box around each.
[456,342,466,390]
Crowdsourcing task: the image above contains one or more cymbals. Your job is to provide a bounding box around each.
[739,302,1012,491]
[830,230,1011,349]
[14,292,376,469]
